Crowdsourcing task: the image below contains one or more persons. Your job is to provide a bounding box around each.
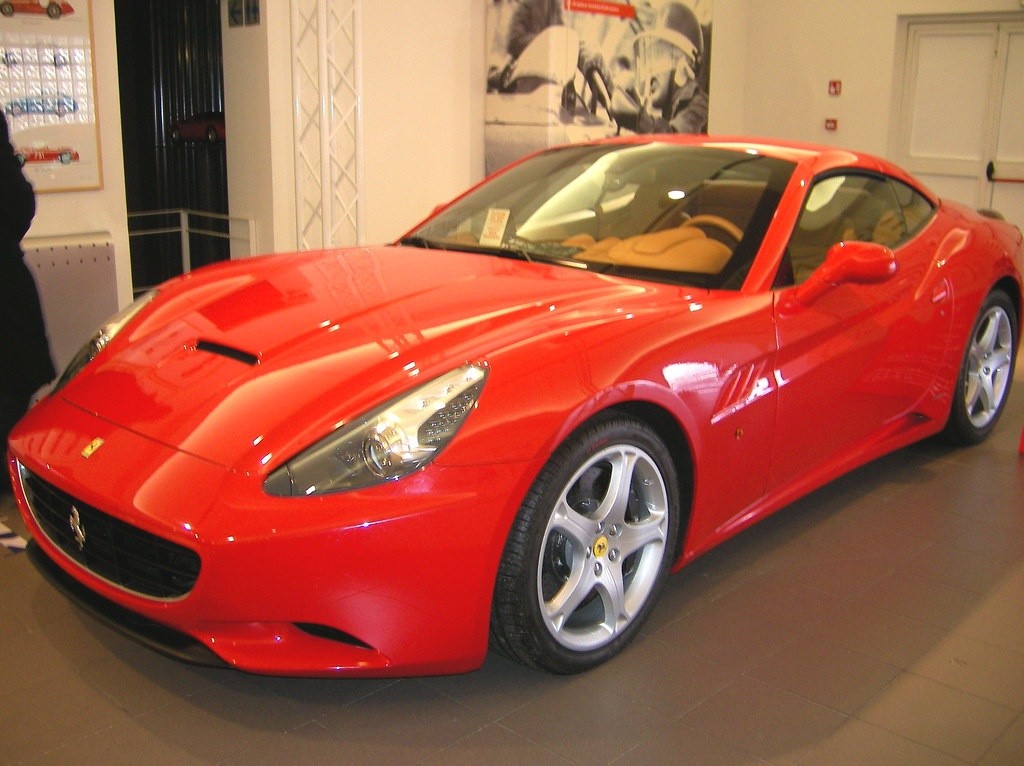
[487,0,709,134]
[0,110,58,552]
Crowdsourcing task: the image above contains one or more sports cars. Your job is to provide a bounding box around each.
[5,134,1024,678]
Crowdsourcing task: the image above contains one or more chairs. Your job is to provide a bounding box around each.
[683,179,794,288]
